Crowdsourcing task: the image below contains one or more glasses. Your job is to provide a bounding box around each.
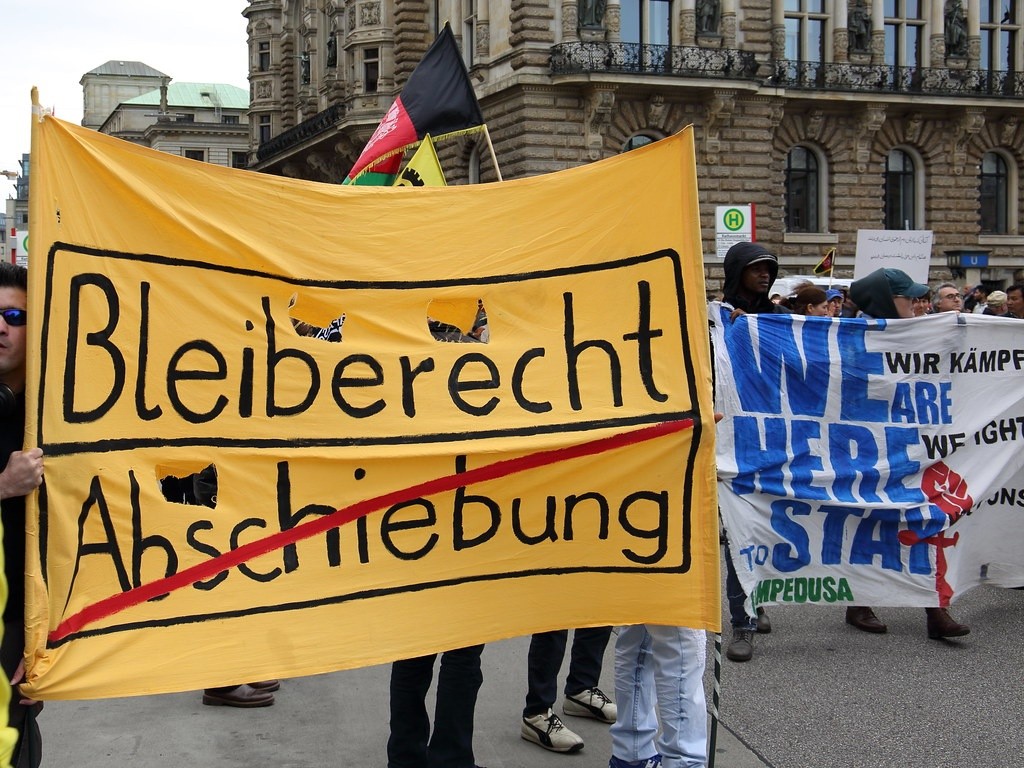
[892,293,912,300]
[0,309,27,327]
[940,292,963,300]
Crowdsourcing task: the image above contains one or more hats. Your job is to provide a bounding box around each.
[745,254,779,268]
[987,290,1008,308]
[883,267,929,298]
[825,289,843,302]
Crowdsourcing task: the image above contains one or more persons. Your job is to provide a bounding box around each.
[719,240,1024,662]
[0,259,712,768]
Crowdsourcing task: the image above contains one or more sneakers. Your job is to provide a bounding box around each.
[609,750,663,768]
[727,627,753,662]
[925,607,971,639]
[522,706,586,755]
[845,605,888,634]
[753,606,773,633]
[563,687,619,723]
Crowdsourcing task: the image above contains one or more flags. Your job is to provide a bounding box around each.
[338,20,486,189]
[813,249,834,276]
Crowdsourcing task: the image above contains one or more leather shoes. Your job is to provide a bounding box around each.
[203,684,275,707]
[247,679,280,692]
